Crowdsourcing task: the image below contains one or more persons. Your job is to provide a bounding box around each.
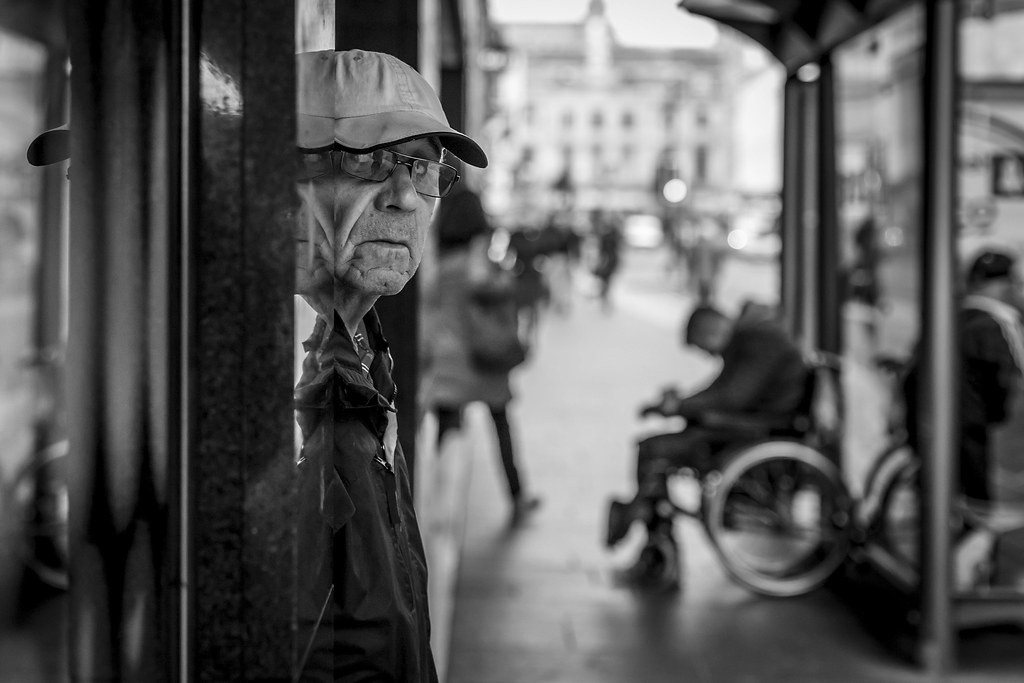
[583,206,622,307]
[827,214,1015,585]
[605,304,817,589]
[297,50,336,682]
[419,189,543,529]
[336,48,489,683]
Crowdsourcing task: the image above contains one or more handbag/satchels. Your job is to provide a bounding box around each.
[458,250,529,376]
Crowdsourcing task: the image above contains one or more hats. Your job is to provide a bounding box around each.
[333,48,488,169]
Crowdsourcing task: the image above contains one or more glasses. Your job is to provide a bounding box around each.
[328,146,461,198]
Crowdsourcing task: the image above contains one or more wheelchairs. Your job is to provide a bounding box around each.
[666,351,856,602]
